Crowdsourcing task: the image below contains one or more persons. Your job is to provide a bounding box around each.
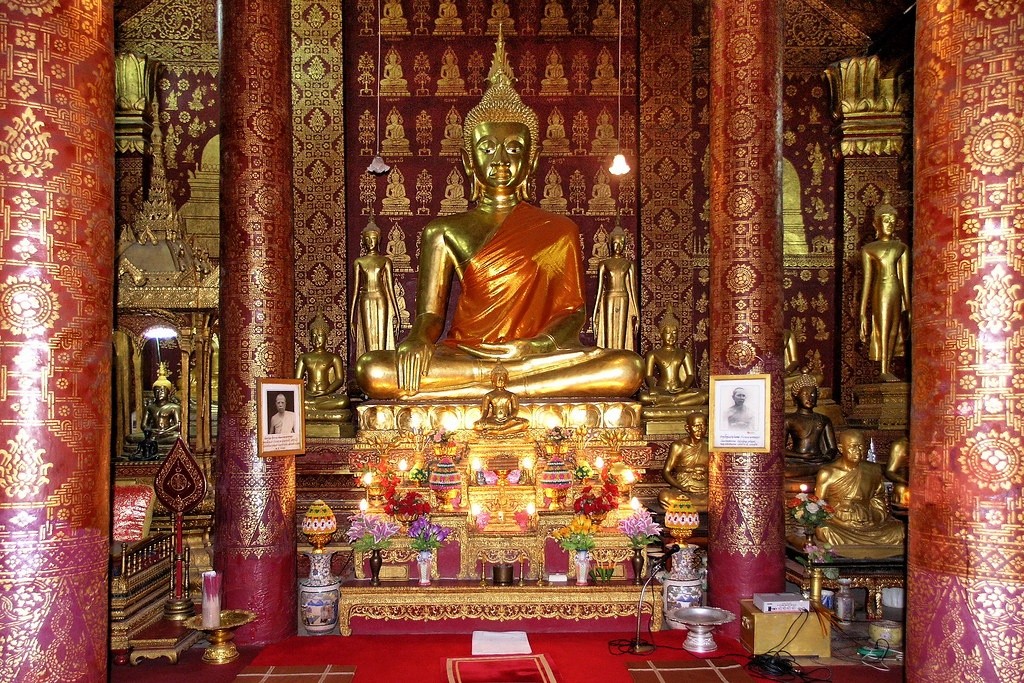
[860,187,913,382]
[657,412,709,512]
[593,208,640,352]
[129,428,160,462]
[125,361,181,444]
[638,298,708,406]
[354,22,647,401]
[812,427,904,548]
[349,197,401,361]
[726,387,754,431]
[270,393,297,436]
[295,301,350,409]
[783,371,842,478]
[883,437,909,507]
[473,358,530,434]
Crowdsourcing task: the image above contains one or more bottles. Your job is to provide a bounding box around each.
[835,578,855,625]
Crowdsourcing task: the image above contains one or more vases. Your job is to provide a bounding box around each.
[298,549,340,636]
[804,532,817,550]
[834,578,855,625]
[368,452,644,586]
[663,543,702,631]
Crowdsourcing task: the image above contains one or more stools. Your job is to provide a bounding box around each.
[129,619,204,665]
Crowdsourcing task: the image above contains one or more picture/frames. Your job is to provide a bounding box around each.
[255,377,307,458]
[708,374,771,454]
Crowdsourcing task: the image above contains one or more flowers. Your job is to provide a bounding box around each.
[346,426,664,550]
[788,492,834,533]
[806,542,841,564]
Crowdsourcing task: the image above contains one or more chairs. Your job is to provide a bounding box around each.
[108,531,179,662]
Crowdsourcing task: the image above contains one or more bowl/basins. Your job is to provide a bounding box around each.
[868,620,903,645]
[820,590,834,610]
[882,587,903,608]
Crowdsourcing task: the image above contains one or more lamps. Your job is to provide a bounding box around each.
[610,0,631,175]
[367,0,390,174]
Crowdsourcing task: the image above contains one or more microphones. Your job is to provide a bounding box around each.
[654,544,681,568]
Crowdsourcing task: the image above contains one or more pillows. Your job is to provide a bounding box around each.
[112,482,156,543]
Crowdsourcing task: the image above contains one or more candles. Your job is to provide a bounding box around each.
[202,571,224,628]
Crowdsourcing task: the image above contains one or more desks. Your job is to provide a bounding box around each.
[781,542,905,625]
